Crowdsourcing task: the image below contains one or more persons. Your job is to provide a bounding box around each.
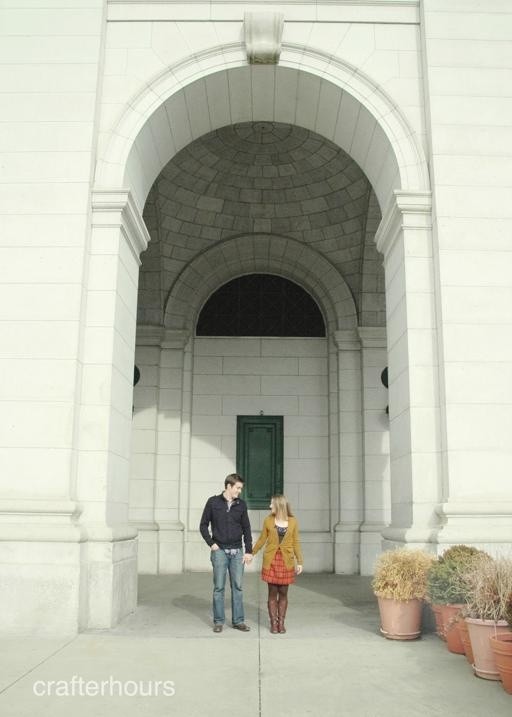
[246,493,303,634]
[199,473,252,632]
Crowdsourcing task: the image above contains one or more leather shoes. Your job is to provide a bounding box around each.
[213,623,223,632]
[232,622,251,632]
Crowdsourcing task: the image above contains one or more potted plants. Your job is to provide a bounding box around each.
[370,543,512,696]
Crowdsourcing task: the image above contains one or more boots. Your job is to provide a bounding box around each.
[268,598,289,635]
